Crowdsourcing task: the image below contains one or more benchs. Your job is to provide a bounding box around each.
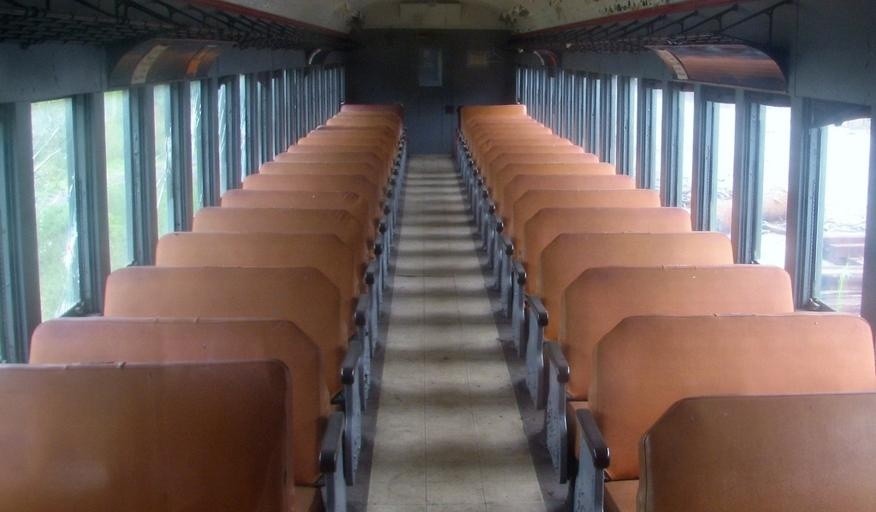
[451,104,875,512]
[1,103,408,512]
